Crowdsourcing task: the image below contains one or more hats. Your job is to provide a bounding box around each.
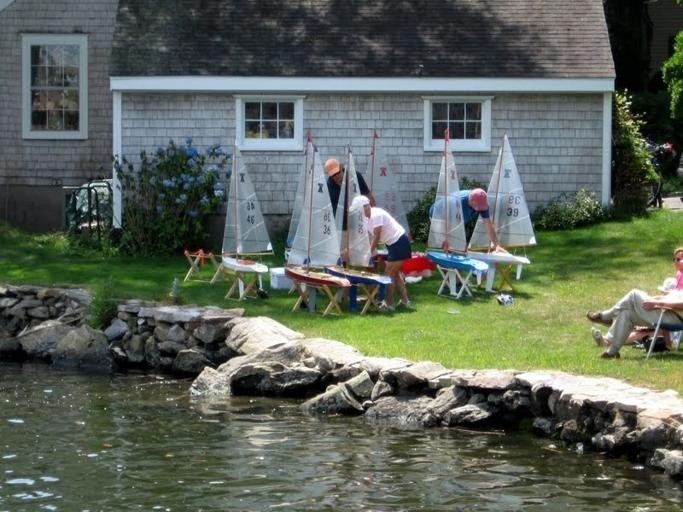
[325,159,339,176]
[470,189,489,211]
[657,277,678,292]
[348,196,370,212]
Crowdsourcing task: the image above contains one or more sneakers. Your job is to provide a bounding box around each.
[376,299,395,311]
[596,352,620,359]
[396,301,411,308]
[587,310,613,327]
[592,328,608,347]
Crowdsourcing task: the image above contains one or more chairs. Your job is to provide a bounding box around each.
[641,302,683,359]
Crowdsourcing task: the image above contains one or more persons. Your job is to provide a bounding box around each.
[425,187,508,253]
[348,195,412,314]
[584,245,683,358]
[319,157,376,249]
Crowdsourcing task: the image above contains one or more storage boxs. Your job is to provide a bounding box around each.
[267,267,297,290]
[397,251,418,271]
[415,251,436,271]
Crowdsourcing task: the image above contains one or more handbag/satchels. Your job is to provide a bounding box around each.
[646,338,666,352]
